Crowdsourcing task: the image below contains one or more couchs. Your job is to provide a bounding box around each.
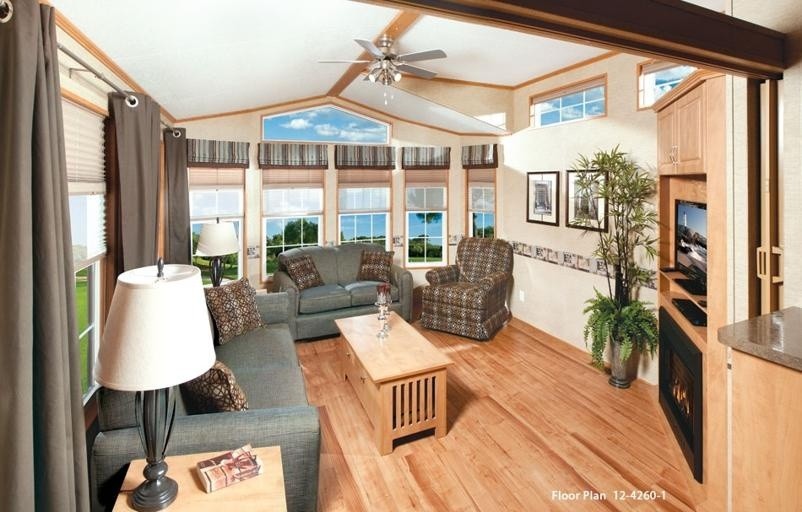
[90,291,320,512]
[273,241,414,342]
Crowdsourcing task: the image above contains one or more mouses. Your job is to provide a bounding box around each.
[698,300,707,307]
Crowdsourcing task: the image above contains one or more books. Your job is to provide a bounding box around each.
[196,443,264,493]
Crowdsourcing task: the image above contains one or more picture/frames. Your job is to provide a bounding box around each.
[526,171,608,234]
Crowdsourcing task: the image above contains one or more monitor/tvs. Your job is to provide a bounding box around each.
[674,198,707,295]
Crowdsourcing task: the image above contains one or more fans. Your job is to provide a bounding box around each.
[318,33,446,80]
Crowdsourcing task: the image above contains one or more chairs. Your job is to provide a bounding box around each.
[421,236,514,340]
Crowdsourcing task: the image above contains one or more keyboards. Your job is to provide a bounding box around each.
[671,298,707,328]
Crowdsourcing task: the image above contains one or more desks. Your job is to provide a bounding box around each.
[716,307,802,512]
[111,445,286,512]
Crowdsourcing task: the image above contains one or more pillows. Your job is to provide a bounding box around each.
[285,249,394,286]
[179,277,264,413]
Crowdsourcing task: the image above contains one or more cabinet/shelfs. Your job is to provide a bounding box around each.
[652,67,727,512]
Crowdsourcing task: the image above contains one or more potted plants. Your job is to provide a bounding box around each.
[569,144,661,388]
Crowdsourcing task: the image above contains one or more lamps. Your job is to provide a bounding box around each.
[192,218,241,287]
[368,68,403,88]
[91,259,217,512]
